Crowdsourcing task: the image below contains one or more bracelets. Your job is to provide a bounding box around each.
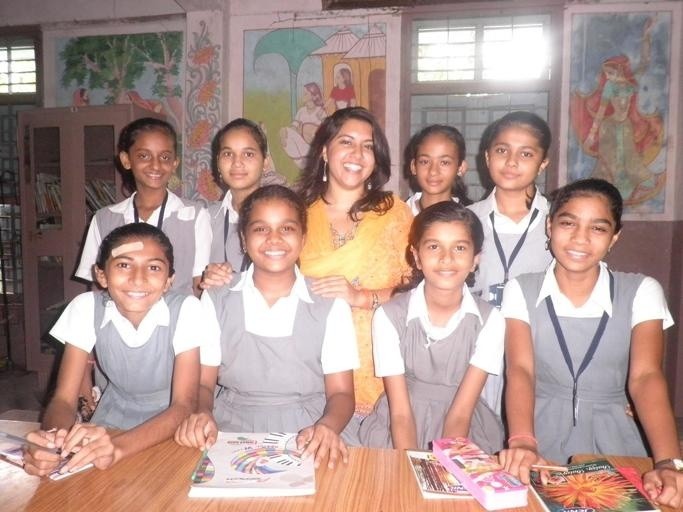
[507,433,537,447]
[368,288,379,312]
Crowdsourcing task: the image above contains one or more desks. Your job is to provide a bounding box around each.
[0,428,683,512]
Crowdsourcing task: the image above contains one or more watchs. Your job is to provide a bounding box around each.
[654,458,683,472]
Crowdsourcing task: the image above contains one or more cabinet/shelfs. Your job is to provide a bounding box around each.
[16,102,166,400]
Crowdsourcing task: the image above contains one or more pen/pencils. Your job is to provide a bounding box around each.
[531,464,568,472]
[0,431,76,460]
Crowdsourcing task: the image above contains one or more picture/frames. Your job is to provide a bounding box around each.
[559,2,682,223]
[228,12,404,202]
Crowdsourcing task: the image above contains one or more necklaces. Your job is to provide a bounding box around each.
[327,222,354,248]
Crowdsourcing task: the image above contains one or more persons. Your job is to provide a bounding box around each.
[325,65,360,111]
[196,105,417,420]
[170,184,363,471]
[71,86,89,105]
[204,116,272,272]
[249,120,286,186]
[22,221,204,479]
[277,81,328,172]
[569,16,665,205]
[73,117,214,424]
[354,202,507,456]
[122,90,168,119]
[499,177,683,510]
[464,110,556,311]
[401,124,473,216]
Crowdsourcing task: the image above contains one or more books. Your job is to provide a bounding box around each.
[186,430,316,496]
[35,172,115,265]
[0,430,94,482]
[406,449,473,498]
[612,466,664,509]
[526,458,654,512]
